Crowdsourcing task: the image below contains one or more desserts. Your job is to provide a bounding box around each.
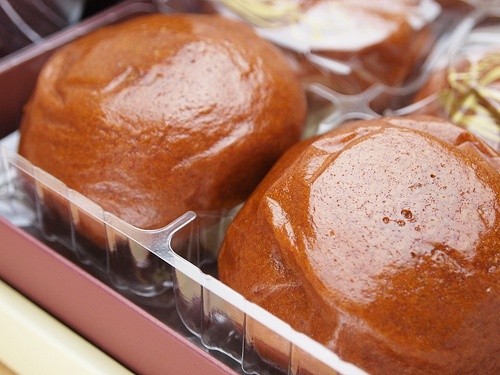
[18,0,500,375]
